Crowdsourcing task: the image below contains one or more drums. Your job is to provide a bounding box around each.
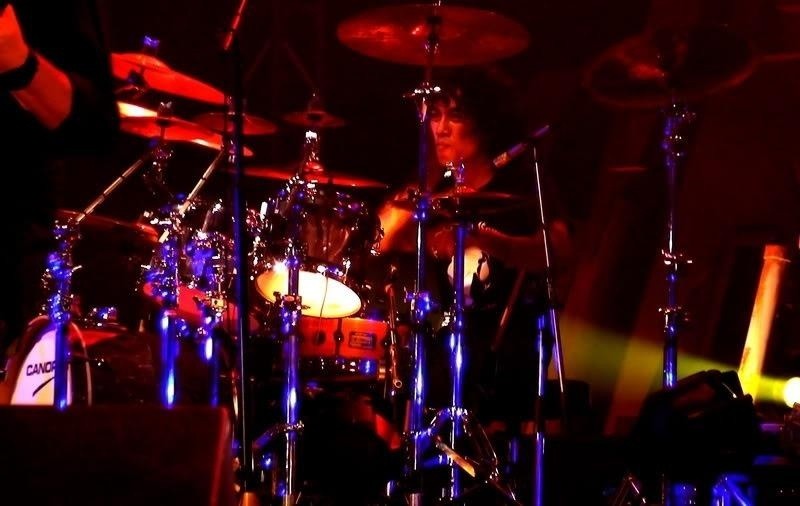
[255,191,409,381]
[0,315,238,421]
[140,196,257,327]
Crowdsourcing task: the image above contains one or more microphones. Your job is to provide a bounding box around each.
[493,126,552,169]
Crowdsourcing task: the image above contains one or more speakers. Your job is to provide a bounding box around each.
[1,402,244,505]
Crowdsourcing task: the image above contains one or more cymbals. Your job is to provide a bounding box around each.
[393,186,539,216]
[579,32,653,111]
[338,5,528,67]
[112,52,389,190]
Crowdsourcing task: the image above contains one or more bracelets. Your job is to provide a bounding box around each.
[1,48,38,94]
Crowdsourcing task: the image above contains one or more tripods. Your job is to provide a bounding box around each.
[420,221,517,506]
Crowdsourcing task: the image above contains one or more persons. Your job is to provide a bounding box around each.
[368,63,555,417]
[1,0,123,141]
